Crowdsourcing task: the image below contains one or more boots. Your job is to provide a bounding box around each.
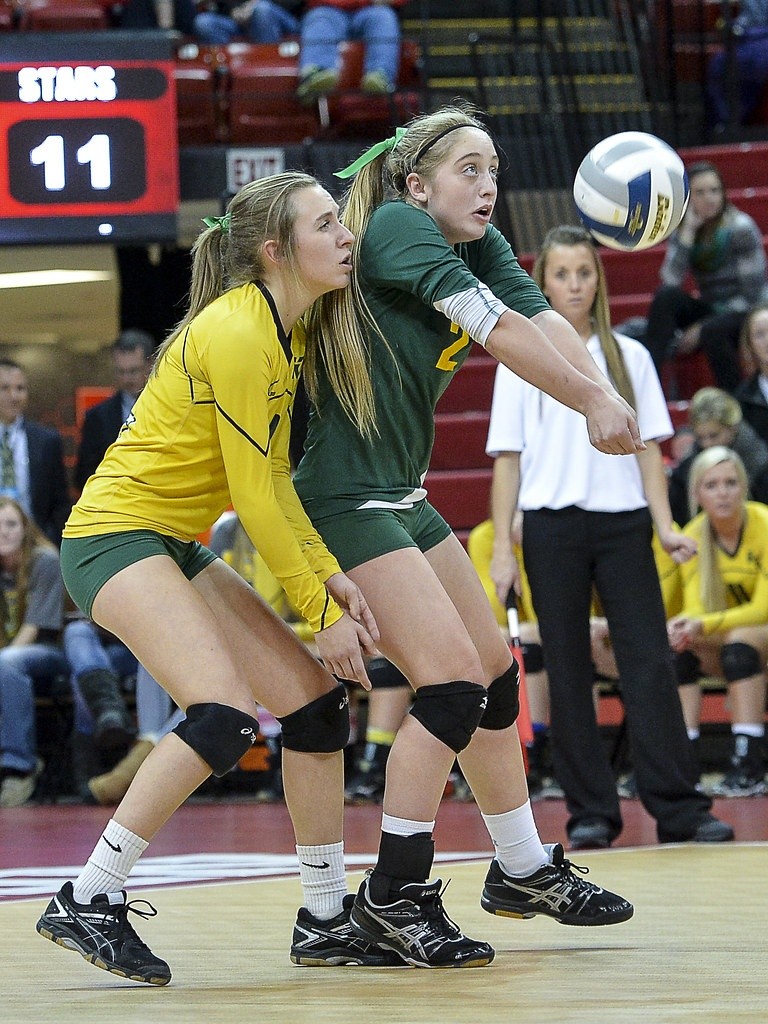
[73,733,108,805]
[87,731,156,807]
[77,667,131,772]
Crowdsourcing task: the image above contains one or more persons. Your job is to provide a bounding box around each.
[36,171,405,982]
[647,160,768,408]
[698,0,768,146]
[293,111,645,967]
[488,228,734,847]
[297,0,402,101]
[0,303,768,807]
[193,0,299,43]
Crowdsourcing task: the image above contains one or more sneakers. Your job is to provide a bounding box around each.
[480,842,634,926]
[35,880,172,986]
[289,893,409,967]
[349,865,495,969]
[344,765,386,806]
[711,771,768,799]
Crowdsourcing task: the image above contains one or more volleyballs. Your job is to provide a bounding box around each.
[572,130,693,253]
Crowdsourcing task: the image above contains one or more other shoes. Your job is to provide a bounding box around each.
[657,810,734,844]
[296,63,339,107]
[0,758,46,808]
[570,817,611,849]
[361,71,392,94]
[541,776,566,800]
[617,774,640,800]
[696,772,728,797]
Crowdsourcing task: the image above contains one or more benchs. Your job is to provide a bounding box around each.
[0,0,768,768]
